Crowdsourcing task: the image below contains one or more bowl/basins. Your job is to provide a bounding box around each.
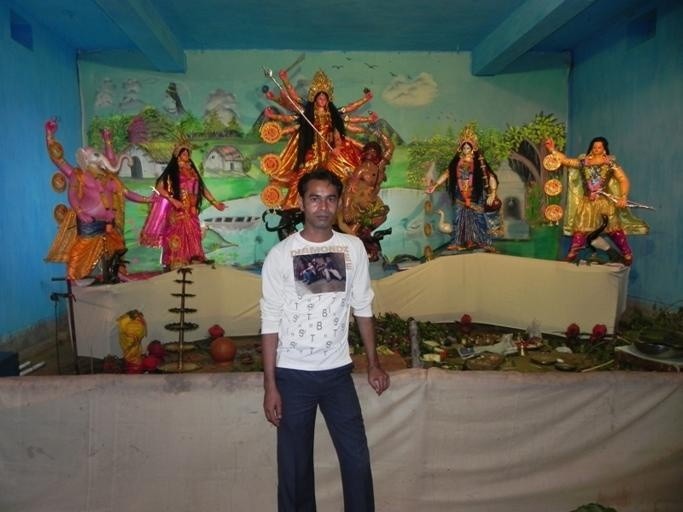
[632,339,683,359]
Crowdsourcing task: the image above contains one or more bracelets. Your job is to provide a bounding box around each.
[143,196,148,203]
[434,182,441,189]
[550,150,557,155]
[490,188,496,193]
[620,193,628,198]
[74,206,82,215]
[210,199,216,206]
[167,196,172,201]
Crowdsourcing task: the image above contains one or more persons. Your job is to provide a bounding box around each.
[425,128,500,252]
[45,116,155,287]
[258,68,395,261]
[258,169,390,511]
[299,255,345,285]
[155,143,228,272]
[545,135,650,266]
